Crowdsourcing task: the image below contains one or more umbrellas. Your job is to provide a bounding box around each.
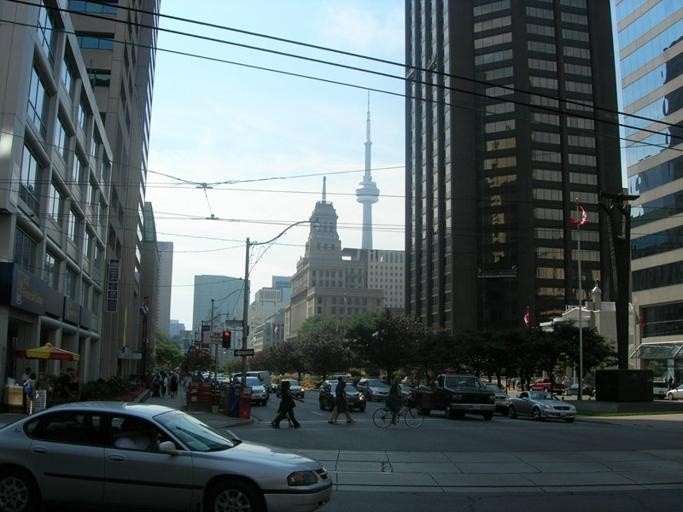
[25,343,80,382]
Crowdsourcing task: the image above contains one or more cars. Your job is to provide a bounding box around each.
[507,378,597,397]
[318,372,426,412]
[653,380,683,400]
[483,383,511,417]
[1,398,334,512]
[192,369,305,406]
[505,392,576,421]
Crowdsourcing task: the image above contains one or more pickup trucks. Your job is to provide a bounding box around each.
[410,371,491,422]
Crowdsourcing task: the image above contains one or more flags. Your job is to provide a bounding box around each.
[569,205,589,225]
[520,305,531,330]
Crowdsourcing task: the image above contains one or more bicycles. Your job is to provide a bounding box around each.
[375,398,426,431]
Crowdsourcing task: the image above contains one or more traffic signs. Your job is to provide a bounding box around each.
[234,349,254,356]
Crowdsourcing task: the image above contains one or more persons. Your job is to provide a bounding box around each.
[271,380,294,429]
[114,416,155,452]
[331,382,357,425]
[327,377,351,424]
[389,375,412,424]
[19,367,82,417]
[142,366,193,400]
[274,380,304,429]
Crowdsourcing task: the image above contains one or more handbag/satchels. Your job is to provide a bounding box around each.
[24,381,33,395]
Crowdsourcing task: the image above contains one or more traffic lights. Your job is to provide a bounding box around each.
[223,332,231,348]
